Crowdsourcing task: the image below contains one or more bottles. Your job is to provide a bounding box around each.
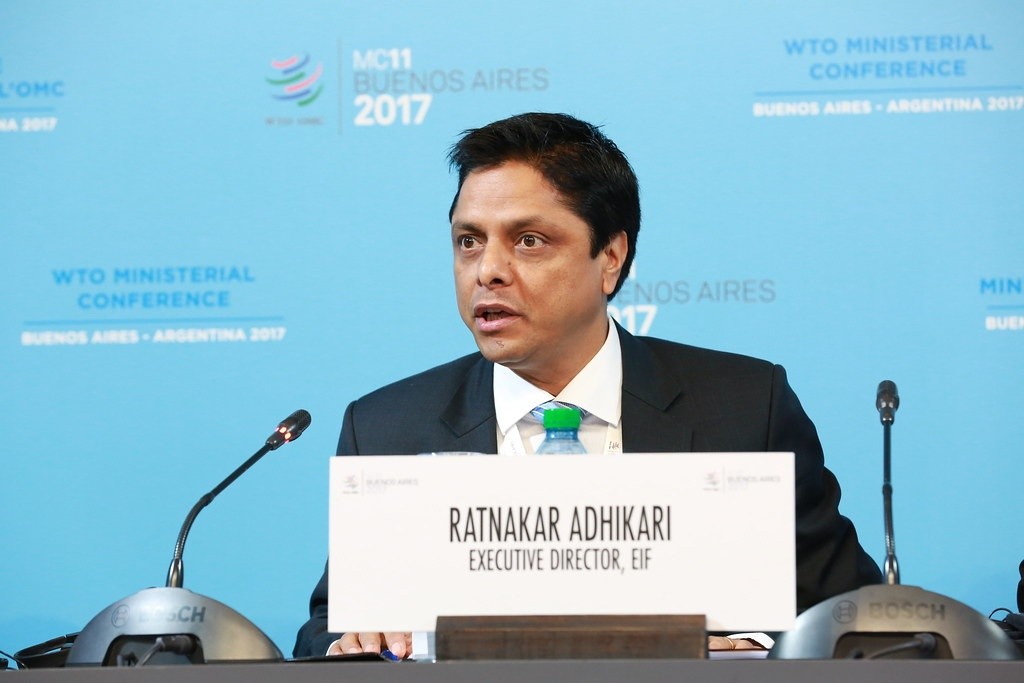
[535,408,586,454]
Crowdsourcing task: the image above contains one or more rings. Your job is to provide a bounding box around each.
[723,636,735,650]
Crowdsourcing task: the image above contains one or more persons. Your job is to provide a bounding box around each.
[291,113,888,659]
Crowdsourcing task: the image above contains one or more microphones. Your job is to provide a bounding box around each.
[766,380,1021,660]
[66,409,312,667]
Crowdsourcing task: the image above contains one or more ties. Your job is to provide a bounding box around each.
[529,401,590,428]
